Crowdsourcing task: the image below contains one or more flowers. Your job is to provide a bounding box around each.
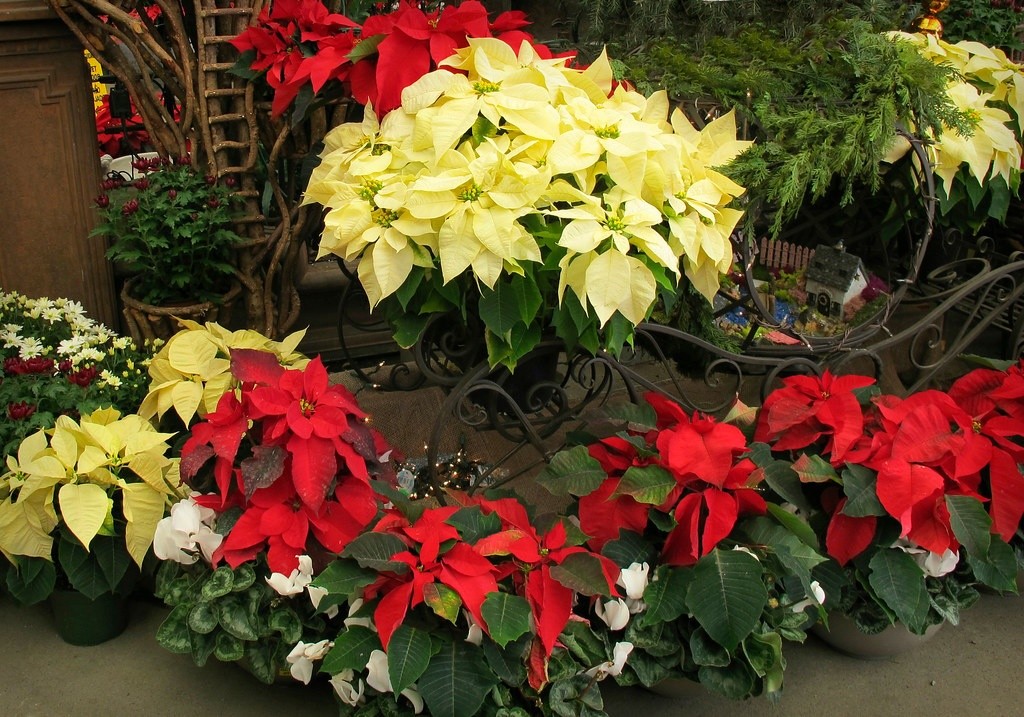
[0,0,1024,717]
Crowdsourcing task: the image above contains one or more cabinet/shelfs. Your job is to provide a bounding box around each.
[0,0,119,332]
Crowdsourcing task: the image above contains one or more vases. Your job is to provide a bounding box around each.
[810,605,947,659]
[426,308,561,417]
[44,582,133,646]
[119,268,243,355]
